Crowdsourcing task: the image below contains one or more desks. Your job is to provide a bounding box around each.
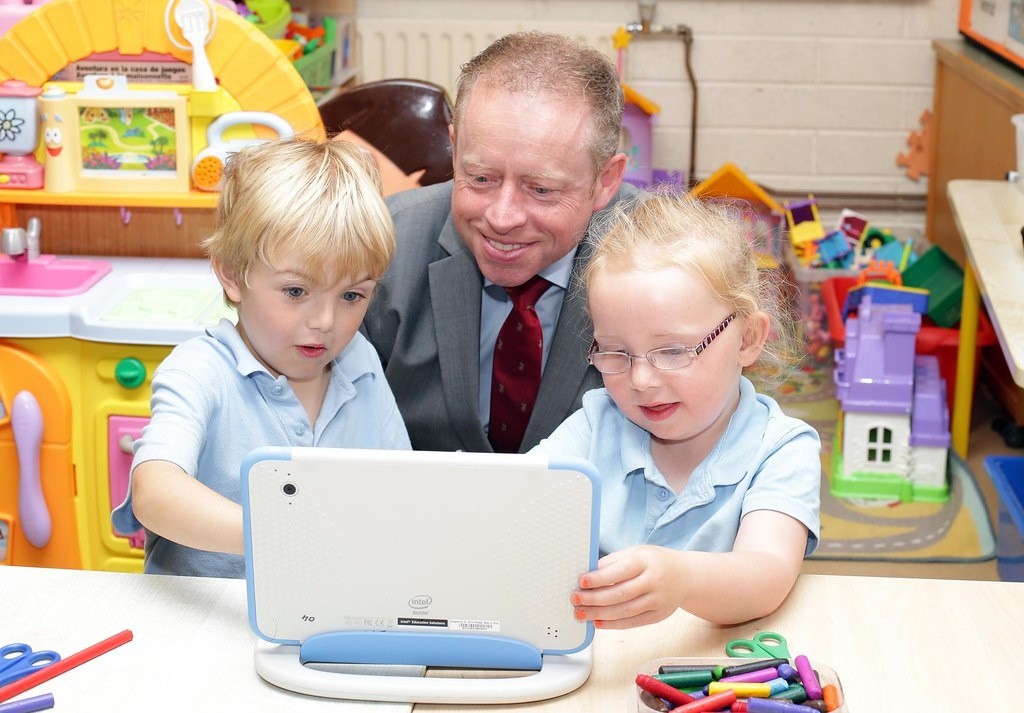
[415,574,1024,713]
[946,176,1024,460]
[0,566,426,713]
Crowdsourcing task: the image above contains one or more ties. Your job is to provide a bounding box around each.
[488,273,556,453]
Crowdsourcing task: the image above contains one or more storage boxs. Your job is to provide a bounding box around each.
[980,455,1024,581]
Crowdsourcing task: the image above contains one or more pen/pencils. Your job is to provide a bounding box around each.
[0,627,135,713]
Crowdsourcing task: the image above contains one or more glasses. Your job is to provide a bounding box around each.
[586,312,739,375]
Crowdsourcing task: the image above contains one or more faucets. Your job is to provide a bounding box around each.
[4,217,44,261]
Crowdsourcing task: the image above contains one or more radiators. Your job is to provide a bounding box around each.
[355,18,625,147]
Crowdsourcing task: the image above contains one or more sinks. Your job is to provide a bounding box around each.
[1,254,112,297]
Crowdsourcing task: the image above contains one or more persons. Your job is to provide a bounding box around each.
[110,140,413,579]
[519,187,824,630]
[359,32,641,454]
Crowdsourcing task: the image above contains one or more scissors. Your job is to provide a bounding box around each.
[0,643,61,688]
[726,631,793,663]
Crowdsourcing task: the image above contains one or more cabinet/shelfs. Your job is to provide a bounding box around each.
[923,36,1024,453]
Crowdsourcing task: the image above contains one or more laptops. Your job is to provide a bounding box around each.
[240,446,603,703]
